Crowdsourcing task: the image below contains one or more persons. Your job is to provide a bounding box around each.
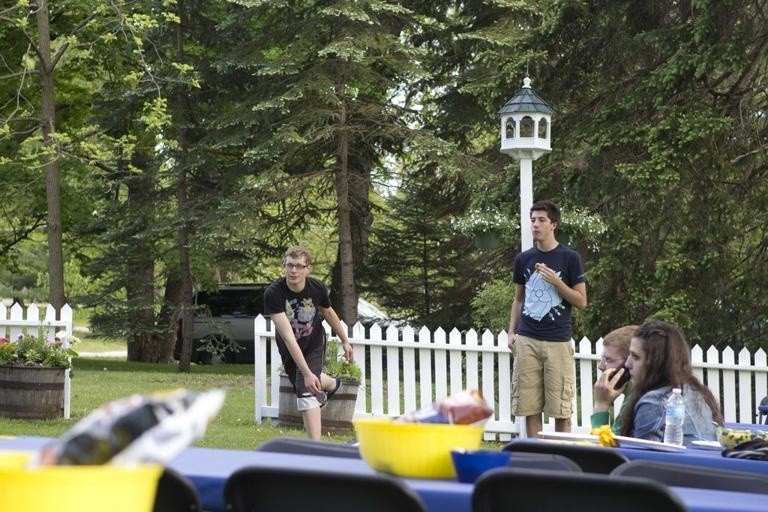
[264,246,355,443]
[507,201,590,437]
[591,319,724,442]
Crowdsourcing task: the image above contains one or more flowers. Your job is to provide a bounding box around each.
[274,337,363,383]
[0,328,80,378]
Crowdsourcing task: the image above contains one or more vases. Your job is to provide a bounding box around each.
[0,365,67,418]
[276,375,359,426]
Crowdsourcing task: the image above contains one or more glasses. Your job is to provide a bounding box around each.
[284,263,307,269]
[600,354,622,364]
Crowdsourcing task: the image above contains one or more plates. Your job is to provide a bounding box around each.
[612,433,688,451]
[693,436,726,452]
[537,428,600,442]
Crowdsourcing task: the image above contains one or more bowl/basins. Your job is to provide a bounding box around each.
[350,416,485,478]
[1,451,163,512]
[450,447,511,483]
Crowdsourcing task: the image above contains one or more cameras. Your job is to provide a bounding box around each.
[610,366,631,387]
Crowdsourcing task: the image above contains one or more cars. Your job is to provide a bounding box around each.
[173,283,430,363]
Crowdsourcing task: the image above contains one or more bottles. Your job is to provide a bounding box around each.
[664,387,685,446]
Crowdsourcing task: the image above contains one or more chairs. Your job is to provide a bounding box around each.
[0,415,768,511]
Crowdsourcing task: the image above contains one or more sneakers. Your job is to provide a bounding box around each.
[320,377,342,409]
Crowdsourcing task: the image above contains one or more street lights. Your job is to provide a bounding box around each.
[497,77,553,253]
